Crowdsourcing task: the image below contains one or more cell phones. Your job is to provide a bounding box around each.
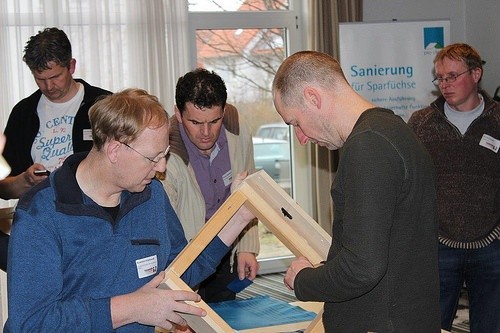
[33,170,51,176]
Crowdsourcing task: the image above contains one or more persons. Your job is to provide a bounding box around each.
[0,27,113,200]
[272,51,442,333]
[408,43,500,333]
[3,89,256,333]
[154,69,260,303]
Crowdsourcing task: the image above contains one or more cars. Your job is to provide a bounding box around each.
[251,123,291,179]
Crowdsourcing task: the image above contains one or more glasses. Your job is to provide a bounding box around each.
[118,139,171,167]
[432,68,471,86]
